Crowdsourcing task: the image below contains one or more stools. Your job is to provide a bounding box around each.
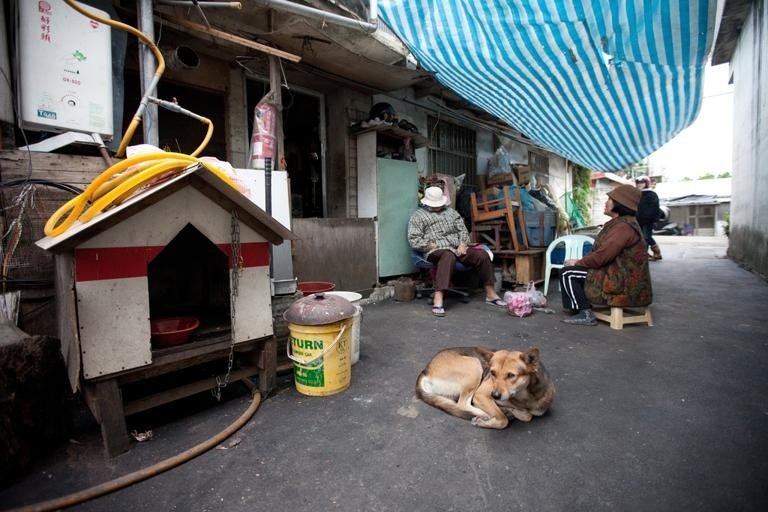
[592,304,654,329]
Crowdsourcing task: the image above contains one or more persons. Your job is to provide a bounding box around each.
[650,178,656,190]
[407,187,509,317]
[635,175,662,261]
[560,183,641,325]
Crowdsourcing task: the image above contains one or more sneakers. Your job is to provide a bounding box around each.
[560,312,598,326]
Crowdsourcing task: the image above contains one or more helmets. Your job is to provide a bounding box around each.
[634,175,651,189]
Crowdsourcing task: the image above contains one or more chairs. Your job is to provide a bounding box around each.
[410,243,474,305]
[472,185,529,250]
[543,233,595,296]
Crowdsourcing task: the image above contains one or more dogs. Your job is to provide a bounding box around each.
[415,346,555,428]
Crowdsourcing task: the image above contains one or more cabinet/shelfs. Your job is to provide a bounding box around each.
[357,127,429,284]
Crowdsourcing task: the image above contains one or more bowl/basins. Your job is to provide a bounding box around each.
[152,317,201,347]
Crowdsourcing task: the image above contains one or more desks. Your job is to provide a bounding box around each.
[490,247,546,290]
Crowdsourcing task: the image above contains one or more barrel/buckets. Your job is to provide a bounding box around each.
[286,315,353,398]
[307,290,364,365]
[296,281,336,298]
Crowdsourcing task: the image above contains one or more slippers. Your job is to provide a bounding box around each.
[433,304,446,318]
[484,298,508,308]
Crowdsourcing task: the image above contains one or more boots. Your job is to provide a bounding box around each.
[651,244,663,260]
[647,251,656,261]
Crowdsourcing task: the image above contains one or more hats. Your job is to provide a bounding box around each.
[420,187,448,209]
[604,183,642,212]
